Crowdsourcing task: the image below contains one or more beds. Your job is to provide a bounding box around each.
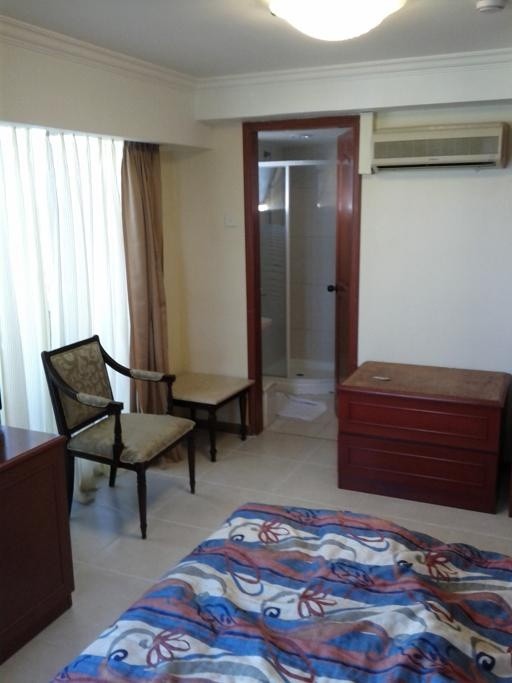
[51,499,511,683]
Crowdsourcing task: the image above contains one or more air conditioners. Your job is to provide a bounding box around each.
[372,118,512,170]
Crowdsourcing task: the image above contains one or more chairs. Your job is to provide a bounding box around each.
[42,334,196,536]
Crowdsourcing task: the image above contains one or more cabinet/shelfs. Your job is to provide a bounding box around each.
[331,360,512,514]
[1,424,79,659]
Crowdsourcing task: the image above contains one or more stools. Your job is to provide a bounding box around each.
[172,370,254,463]
